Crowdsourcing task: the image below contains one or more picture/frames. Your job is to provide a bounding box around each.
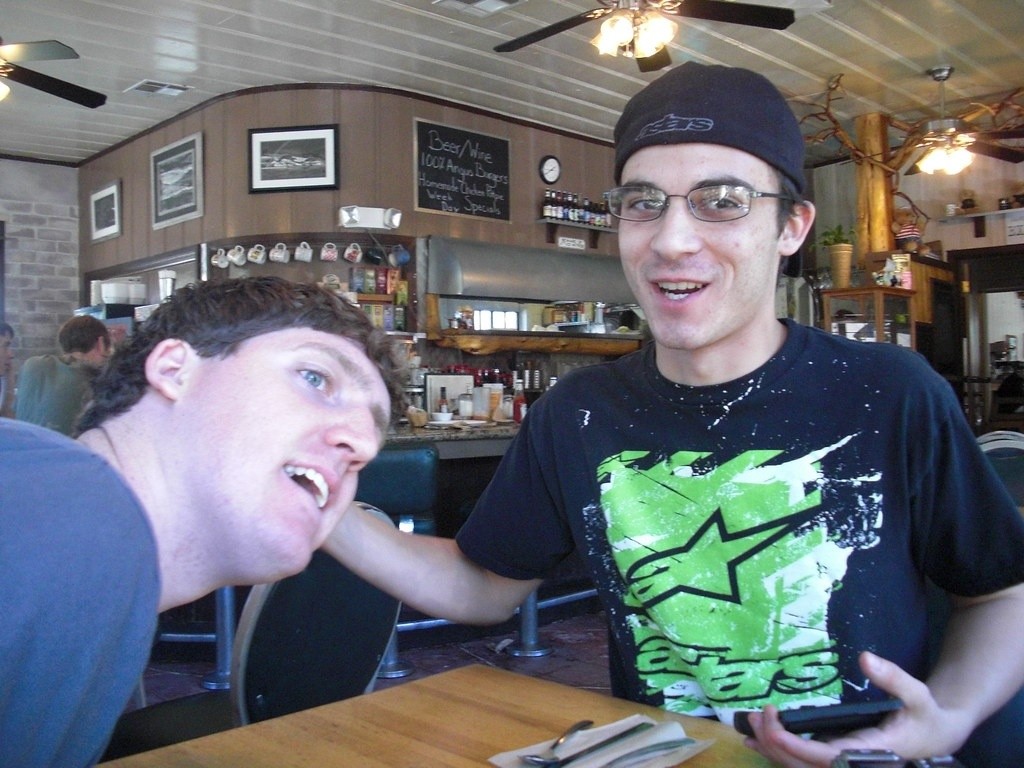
[248,123,341,193]
[150,131,204,231]
[87,178,122,246]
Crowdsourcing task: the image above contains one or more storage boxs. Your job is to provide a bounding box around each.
[350,267,400,294]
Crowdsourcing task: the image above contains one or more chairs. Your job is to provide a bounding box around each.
[976,431,1024,507]
[103,500,403,761]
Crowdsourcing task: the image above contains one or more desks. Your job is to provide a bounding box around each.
[94,663,779,768]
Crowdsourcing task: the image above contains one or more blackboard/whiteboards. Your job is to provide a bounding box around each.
[413,117,513,224]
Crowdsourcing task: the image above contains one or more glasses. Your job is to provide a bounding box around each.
[603,184,794,222]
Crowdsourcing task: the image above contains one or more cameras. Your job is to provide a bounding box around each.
[831,748,964,768]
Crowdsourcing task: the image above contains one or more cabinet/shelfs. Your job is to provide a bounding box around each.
[206,232,418,344]
[427,218,643,356]
[819,286,917,351]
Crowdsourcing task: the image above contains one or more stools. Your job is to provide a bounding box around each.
[356,444,440,676]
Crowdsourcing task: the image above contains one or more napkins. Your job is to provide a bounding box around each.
[486,714,716,768]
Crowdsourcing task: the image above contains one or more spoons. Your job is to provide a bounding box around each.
[522,720,594,764]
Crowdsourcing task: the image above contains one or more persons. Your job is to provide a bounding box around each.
[317,61,1024,768]
[0,278,416,768]
[0,316,113,439]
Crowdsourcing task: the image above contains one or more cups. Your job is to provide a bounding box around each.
[159,278,176,304]
[211,248,229,268]
[471,386,489,420]
[268,242,289,263]
[294,242,313,262]
[483,383,503,420]
[895,313,909,323]
[344,242,363,263]
[945,204,959,216]
[246,244,266,264]
[226,245,246,266]
[320,243,338,260]
[367,248,384,265]
[388,244,411,268]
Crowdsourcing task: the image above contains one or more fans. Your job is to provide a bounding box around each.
[890,66,1024,175]
[494,0,795,73]
[0,36,107,109]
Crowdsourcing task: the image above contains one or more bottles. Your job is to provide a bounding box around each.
[542,189,611,227]
[439,386,447,412]
[894,271,901,287]
[458,369,558,423]
[999,199,1008,209]
[542,306,566,324]
[903,270,911,288]
[456,305,474,331]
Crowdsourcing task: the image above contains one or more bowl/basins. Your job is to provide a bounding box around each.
[1014,194,1024,206]
[432,412,453,421]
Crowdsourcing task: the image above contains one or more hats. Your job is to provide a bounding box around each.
[614,61,808,277]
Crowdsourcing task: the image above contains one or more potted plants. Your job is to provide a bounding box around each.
[809,224,857,288]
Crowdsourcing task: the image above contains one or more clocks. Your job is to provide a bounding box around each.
[539,155,562,185]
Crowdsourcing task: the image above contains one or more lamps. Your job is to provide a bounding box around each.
[916,135,975,175]
[600,0,678,59]
[339,205,402,230]
[0,63,15,102]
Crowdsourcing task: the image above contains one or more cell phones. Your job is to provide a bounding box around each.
[734,699,905,737]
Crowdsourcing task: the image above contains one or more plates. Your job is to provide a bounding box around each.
[462,419,486,426]
[429,421,457,428]
